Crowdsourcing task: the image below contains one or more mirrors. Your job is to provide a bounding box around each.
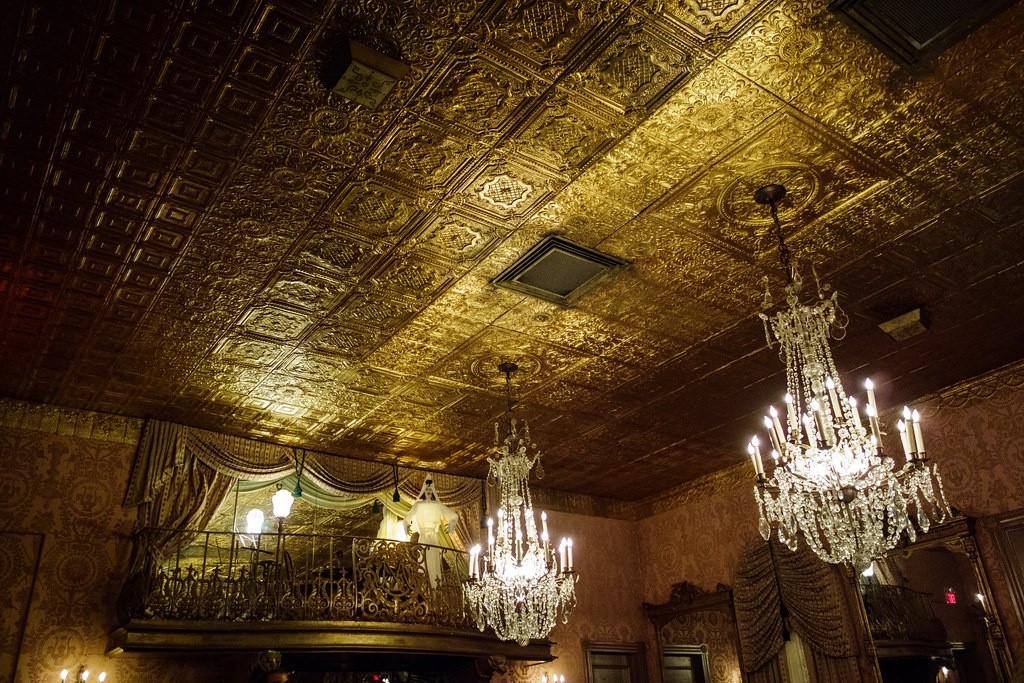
[139,418,487,621]
[643,580,746,683]
[846,507,1016,683]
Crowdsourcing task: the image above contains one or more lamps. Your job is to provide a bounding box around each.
[746,184,953,571]
[462,361,577,644]
